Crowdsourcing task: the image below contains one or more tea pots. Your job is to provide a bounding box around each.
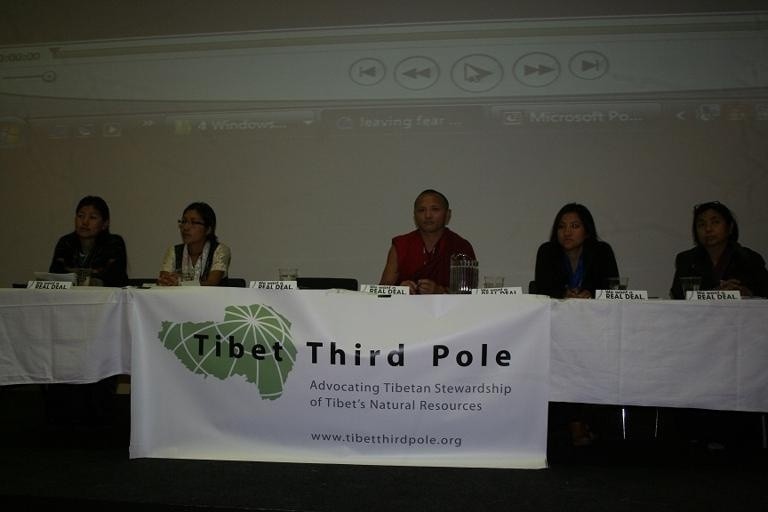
[449,248,479,294]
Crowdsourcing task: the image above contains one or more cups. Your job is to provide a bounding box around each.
[484,278,504,288]
[278,267,298,281]
[608,278,629,289]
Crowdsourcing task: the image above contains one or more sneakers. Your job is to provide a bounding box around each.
[571,432,730,459]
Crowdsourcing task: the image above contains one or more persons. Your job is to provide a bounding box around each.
[534,202,620,447]
[670,201,768,451]
[45,196,128,444]
[157,199,231,286]
[376,187,479,295]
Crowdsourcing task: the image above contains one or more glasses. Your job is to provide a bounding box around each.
[177,219,207,230]
[693,200,721,214]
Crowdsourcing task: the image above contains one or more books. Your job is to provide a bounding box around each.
[33,271,80,285]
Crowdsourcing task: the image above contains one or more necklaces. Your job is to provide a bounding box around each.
[422,246,435,266]
[186,248,201,258]
